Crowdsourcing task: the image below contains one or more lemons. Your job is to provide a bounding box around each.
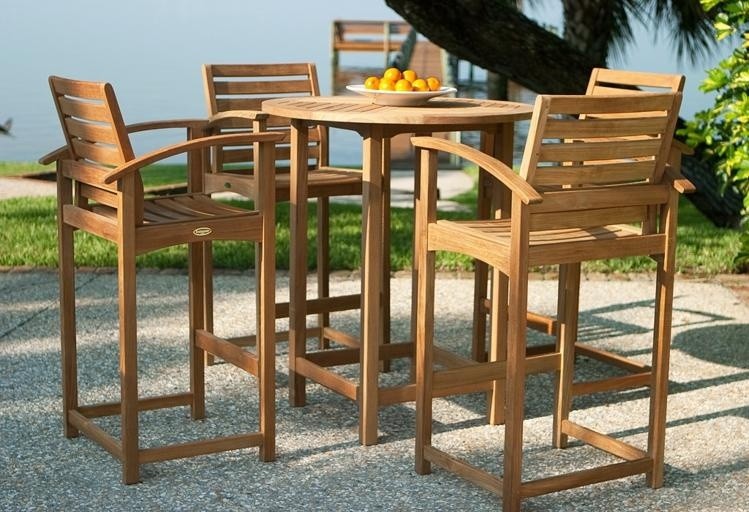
[365,67,440,92]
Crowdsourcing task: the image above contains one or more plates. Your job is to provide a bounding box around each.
[345,84,458,107]
